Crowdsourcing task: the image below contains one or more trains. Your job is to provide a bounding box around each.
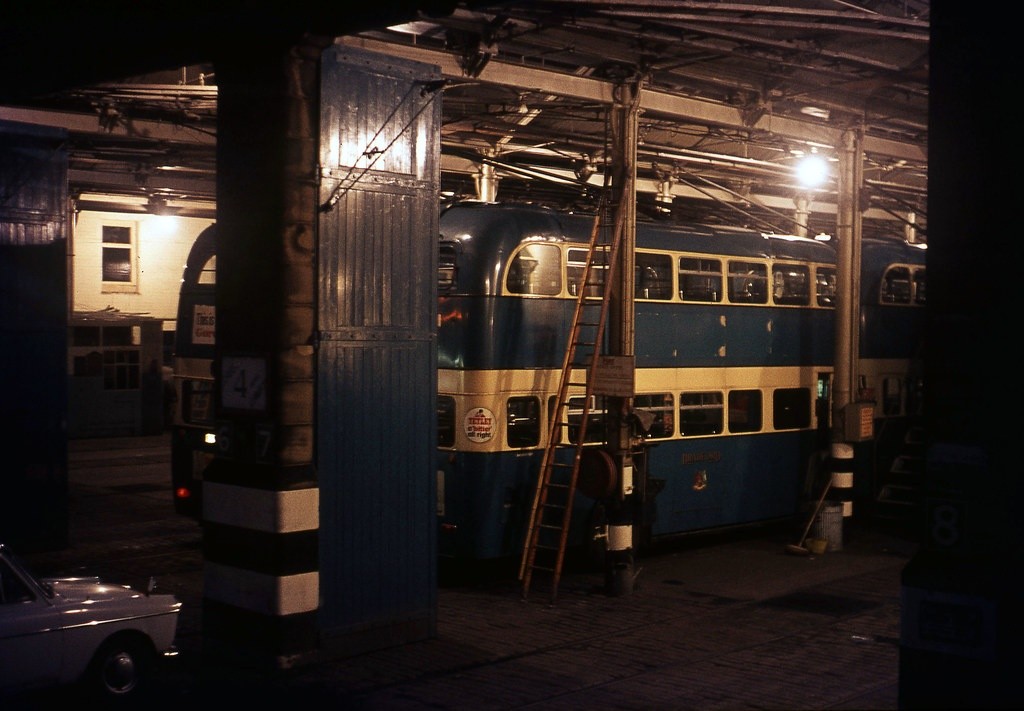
[171,187,927,586]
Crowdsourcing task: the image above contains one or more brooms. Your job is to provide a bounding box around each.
[781,479,833,557]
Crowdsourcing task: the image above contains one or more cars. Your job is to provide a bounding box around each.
[0,543,181,709]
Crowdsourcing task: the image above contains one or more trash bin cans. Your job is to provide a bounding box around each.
[806,498,845,554]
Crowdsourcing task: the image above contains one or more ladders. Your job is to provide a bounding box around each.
[515,187,630,598]
